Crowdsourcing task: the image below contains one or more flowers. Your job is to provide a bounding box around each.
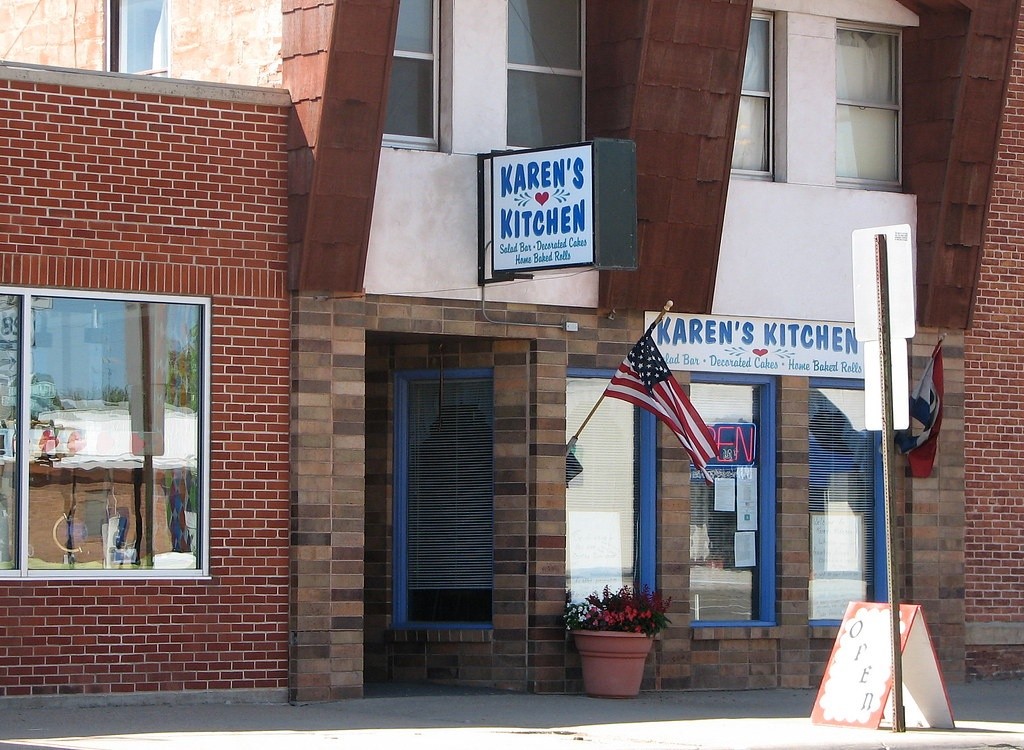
[562,582,673,640]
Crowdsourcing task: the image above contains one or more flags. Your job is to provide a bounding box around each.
[603,317,721,486]
[895,337,944,479]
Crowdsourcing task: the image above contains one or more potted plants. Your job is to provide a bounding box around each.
[568,629,656,700]
